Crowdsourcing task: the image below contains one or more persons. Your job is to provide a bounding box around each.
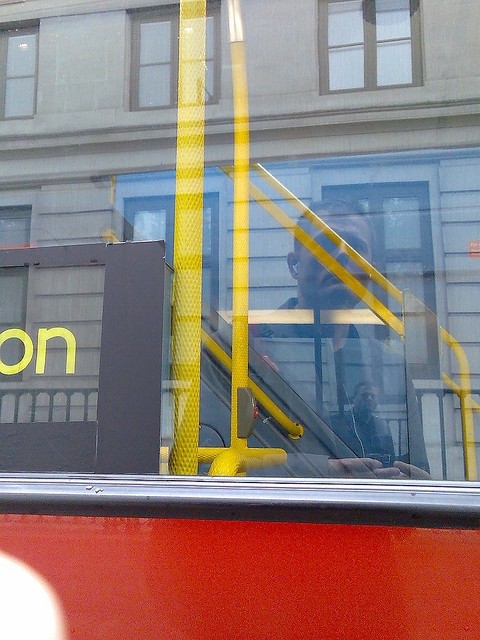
[329,381,396,469]
[199,194,431,481]
